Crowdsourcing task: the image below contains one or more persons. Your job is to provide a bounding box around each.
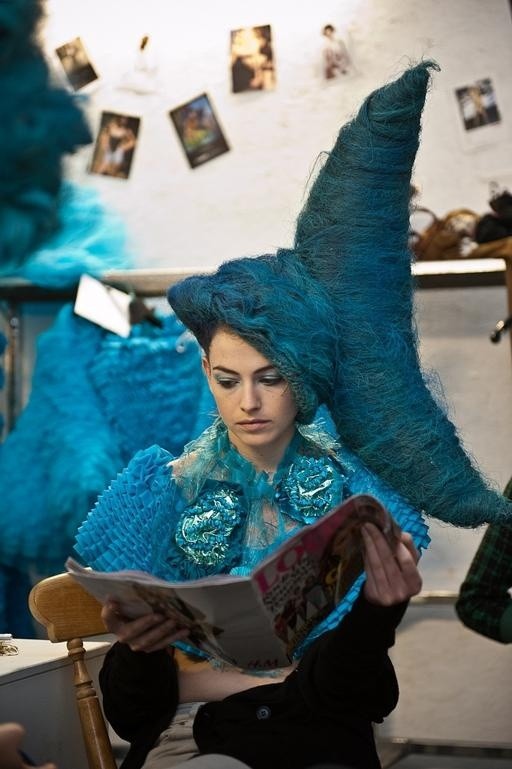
[272,504,390,662]
[179,106,221,155]
[93,114,138,180]
[73,250,431,768]
[323,24,357,82]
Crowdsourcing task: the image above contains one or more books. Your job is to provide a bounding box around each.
[63,491,402,673]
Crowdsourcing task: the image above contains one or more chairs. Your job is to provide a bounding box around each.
[29,566,126,768]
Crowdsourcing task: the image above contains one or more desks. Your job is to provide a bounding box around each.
[0,637,112,768]
